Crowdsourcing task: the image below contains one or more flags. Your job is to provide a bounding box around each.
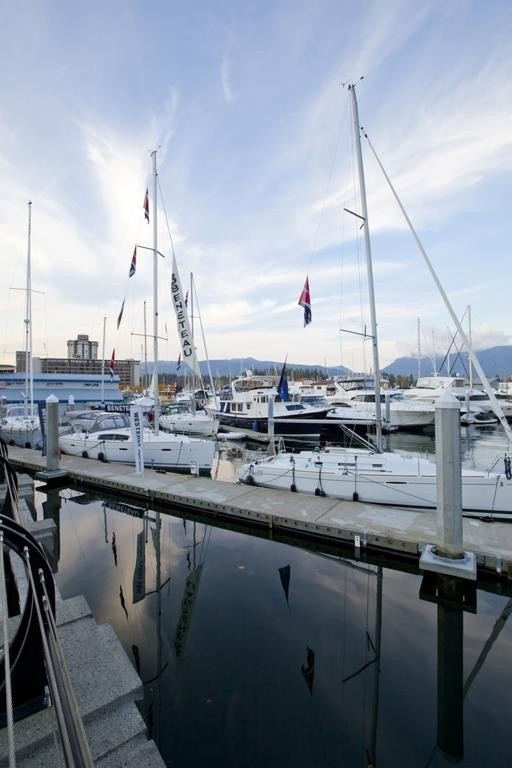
[109,347,115,383]
[129,245,136,277]
[299,275,312,329]
[175,352,182,371]
[117,297,125,330]
[142,185,150,224]
[276,362,288,401]
